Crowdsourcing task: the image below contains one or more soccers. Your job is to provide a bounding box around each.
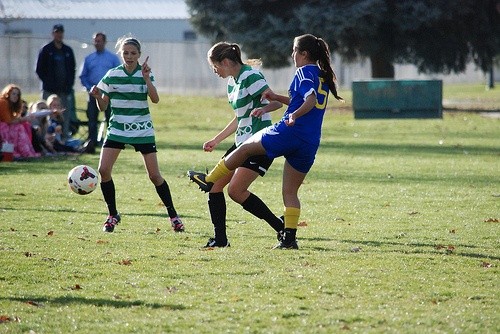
[68,165,98,196]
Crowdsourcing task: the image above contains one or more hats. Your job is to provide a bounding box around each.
[52,24,64,32]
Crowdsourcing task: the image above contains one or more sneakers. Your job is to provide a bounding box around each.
[104,213,120,232]
[204,238,230,247]
[271,241,298,249]
[186,169,214,193]
[171,216,184,232]
[277,215,284,241]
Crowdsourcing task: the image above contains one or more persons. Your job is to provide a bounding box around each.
[202,42,283,249]
[36,24,76,139]
[79,33,122,153]
[0,84,92,157]
[88,38,185,233]
[187,34,343,249]
